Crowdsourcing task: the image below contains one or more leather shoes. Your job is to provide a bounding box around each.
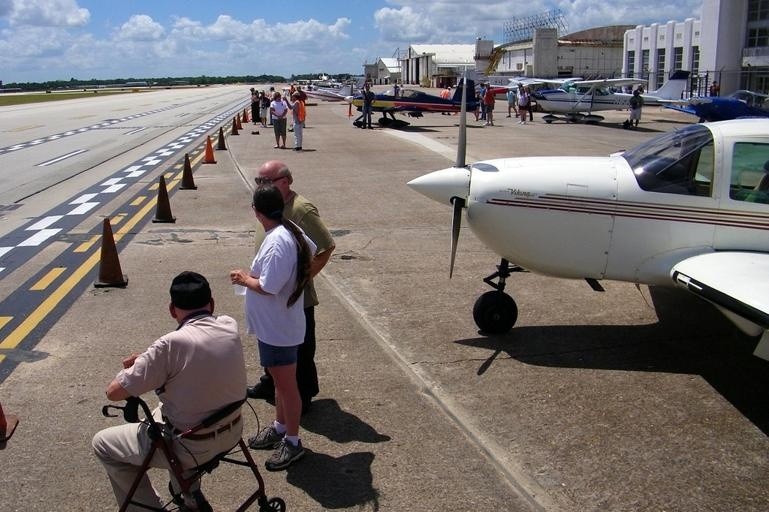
[241,379,274,401]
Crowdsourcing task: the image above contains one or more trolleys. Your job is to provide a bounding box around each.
[96,376,288,512]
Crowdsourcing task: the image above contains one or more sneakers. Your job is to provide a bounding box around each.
[271,144,305,153]
[258,123,268,129]
[360,125,376,131]
[472,114,534,127]
[264,435,307,473]
[246,424,288,451]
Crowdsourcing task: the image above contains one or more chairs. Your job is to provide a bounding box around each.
[118,395,287,512]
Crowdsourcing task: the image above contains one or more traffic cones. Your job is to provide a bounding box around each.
[203,106,250,166]
[93,219,129,288]
[177,151,197,191]
[152,174,178,226]
[0,402,23,444]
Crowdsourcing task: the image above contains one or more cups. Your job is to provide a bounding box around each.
[234,284,247,295]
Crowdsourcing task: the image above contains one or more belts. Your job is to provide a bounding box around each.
[158,412,253,444]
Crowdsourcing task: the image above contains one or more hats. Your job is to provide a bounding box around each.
[291,91,302,97]
[259,89,266,94]
[169,270,212,312]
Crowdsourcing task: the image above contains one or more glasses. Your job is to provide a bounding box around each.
[253,175,290,186]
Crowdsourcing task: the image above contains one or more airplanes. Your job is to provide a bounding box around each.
[401,69,769,366]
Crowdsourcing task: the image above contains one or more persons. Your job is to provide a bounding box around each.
[629,90,644,127]
[249,85,307,153]
[710,80,720,96]
[246,159,335,406]
[90,270,249,512]
[350,77,534,130]
[637,84,644,94]
[229,181,319,471]
